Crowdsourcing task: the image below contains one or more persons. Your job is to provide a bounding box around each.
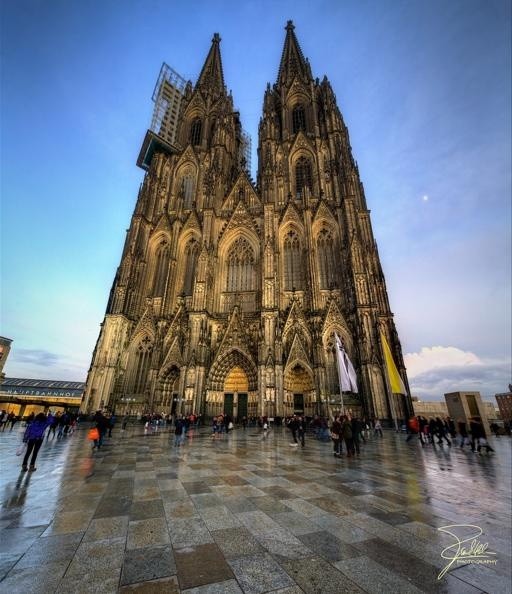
[0,405,502,470]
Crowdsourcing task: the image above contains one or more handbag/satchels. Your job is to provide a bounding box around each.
[330,432,340,441]
[86,426,100,441]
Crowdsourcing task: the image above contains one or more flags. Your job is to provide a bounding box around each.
[333,332,359,393]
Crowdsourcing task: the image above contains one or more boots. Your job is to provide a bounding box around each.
[21,464,28,472]
[28,465,37,472]
